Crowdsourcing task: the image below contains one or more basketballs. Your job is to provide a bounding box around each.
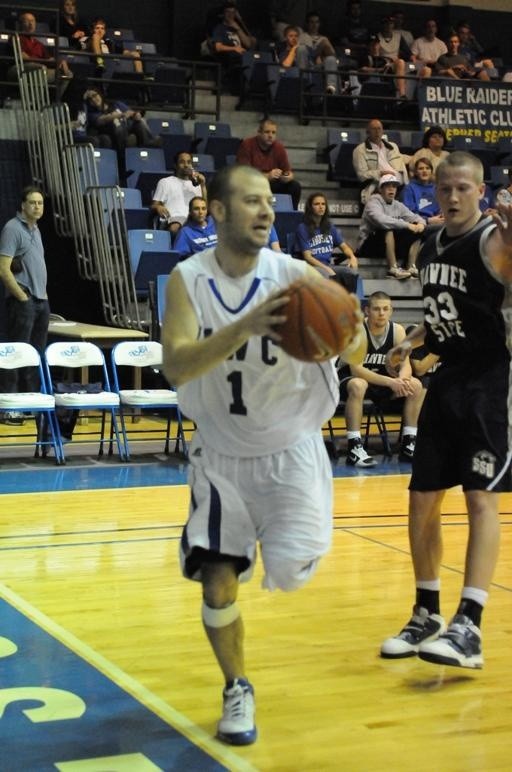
[269,277,361,362]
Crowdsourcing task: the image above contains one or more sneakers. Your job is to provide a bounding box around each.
[216,679,257,745]
[0,412,26,425]
[381,605,445,659]
[385,264,420,279]
[346,444,377,467]
[399,441,415,462]
[418,613,483,669]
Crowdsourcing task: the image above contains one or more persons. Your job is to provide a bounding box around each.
[0,185,50,426]
[336,291,423,469]
[380,148,512,671]
[404,320,443,402]
[0,1,512,284]
[158,165,368,746]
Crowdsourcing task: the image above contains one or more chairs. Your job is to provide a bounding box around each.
[0,341,61,466]
[36,341,124,465]
[0,1,512,336]
[108,340,189,463]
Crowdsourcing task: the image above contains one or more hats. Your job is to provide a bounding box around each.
[379,174,400,187]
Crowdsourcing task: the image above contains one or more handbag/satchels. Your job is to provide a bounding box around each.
[10,255,23,274]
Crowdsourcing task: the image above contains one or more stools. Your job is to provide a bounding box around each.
[327,398,390,463]
[362,396,404,459]
